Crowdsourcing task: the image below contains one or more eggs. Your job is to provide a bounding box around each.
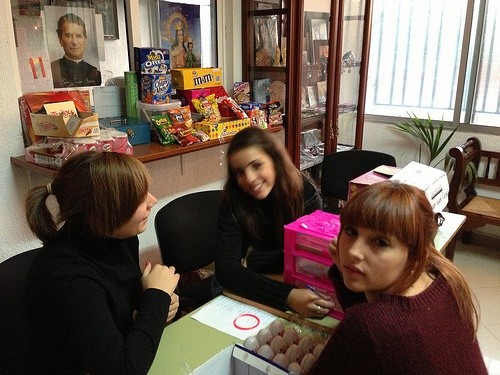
[244,319,324,375]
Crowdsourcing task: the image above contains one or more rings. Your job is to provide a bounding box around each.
[318,306,321,313]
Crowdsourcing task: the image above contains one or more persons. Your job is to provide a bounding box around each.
[214,128,335,320]
[23,152,179,375]
[306,180,490,375]
[51,13,102,90]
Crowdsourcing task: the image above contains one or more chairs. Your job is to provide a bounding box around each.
[445,137,500,262]
[0,150,397,375]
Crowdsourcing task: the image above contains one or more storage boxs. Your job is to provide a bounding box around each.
[284,161,449,320]
[99,47,251,146]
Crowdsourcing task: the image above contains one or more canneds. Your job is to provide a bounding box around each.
[47,129,128,156]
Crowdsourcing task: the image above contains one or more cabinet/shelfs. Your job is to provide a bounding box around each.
[241,0,374,172]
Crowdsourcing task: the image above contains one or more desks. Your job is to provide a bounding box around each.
[147,211,466,375]
[9,126,285,274]
